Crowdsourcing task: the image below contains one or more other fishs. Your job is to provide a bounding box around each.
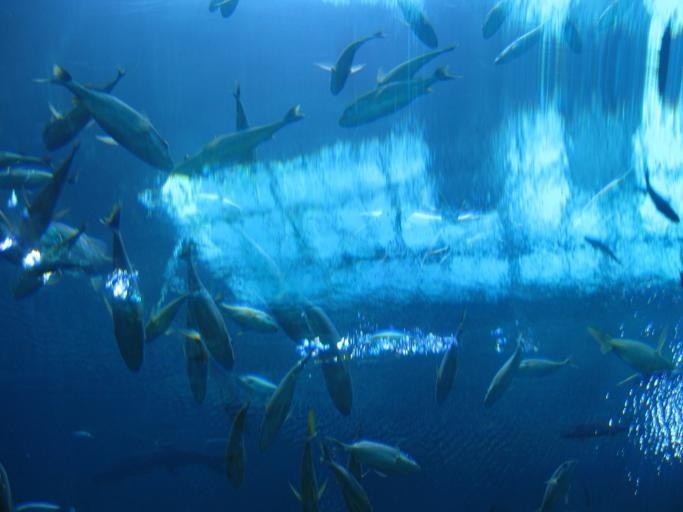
[0,0,683,512]
